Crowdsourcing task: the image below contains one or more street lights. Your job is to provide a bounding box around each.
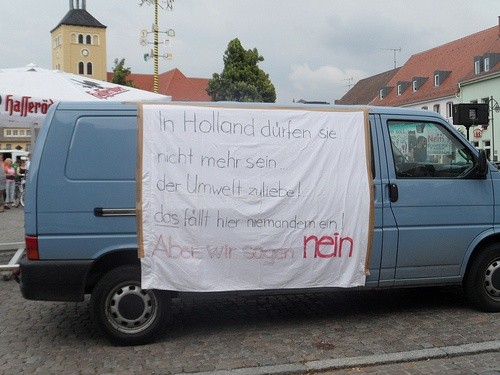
[481,97,500,161]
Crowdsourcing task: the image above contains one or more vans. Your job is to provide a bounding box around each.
[19,100,500,347]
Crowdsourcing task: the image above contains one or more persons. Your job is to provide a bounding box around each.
[0,153,29,214]
[409,136,427,163]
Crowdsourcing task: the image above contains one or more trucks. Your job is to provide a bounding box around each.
[0,149,30,166]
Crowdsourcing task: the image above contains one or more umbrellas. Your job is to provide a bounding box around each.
[0,62,173,151]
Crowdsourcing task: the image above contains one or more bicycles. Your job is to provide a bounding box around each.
[4,172,27,208]
